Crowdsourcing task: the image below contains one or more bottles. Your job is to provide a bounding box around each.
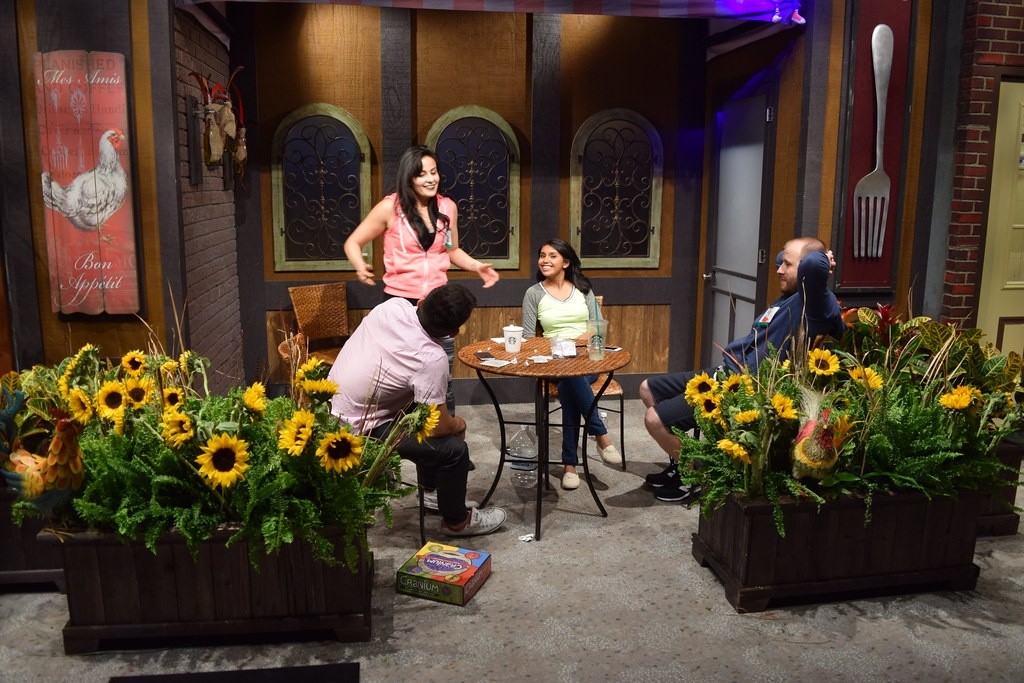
[600,411,608,428]
[508,424,543,487]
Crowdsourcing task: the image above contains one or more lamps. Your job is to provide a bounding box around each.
[184,62,247,193]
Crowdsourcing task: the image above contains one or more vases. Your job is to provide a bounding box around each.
[1,485,68,590]
[37,517,378,655]
[976,437,1024,537]
[691,485,980,615]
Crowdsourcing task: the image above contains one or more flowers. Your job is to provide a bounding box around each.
[672,274,1024,538]
[0,278,440,574]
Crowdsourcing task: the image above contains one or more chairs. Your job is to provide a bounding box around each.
[288,280,351,365]
[277,332,427,548]
[534,295,626,469]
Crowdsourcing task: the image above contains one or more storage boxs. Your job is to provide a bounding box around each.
[396,541,492,607]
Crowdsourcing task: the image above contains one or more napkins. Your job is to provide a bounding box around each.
[490,337,527,343]
[481,359,512,369]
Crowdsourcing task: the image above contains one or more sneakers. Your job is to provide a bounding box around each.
[655,474,702,501]
[646,455,680,487]
[415,489,479,510]
[437,507,507,535]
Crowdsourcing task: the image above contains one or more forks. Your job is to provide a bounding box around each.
[852,23,893,257]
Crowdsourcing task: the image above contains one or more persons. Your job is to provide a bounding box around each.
[522,240,621,490]
[639,237,842,501]
[328,283,507,536]
[344,149,499,469]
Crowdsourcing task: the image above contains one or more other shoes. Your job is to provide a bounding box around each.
[562,470,580,489]
[596,442,622,464]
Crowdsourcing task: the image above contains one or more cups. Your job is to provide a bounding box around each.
[502,326,523,353]
[585,320,610,360]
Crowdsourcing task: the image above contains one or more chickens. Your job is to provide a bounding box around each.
[41,128,127,244]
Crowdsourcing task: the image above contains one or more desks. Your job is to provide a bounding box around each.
[457,337,632,542]
